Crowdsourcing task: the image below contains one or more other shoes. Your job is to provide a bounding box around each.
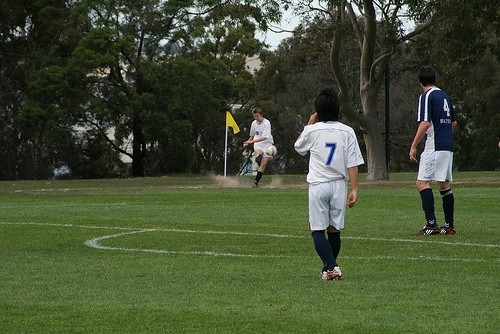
[256,153,262,165]
[252,182,259,187]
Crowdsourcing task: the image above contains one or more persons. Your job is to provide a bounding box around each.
[409,67,458,235]
[294,89,366,280]
[243,108,277,188]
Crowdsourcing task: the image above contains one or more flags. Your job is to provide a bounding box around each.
[226,111,240,135]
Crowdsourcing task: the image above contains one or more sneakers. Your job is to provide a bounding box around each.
[439,225,456,235]
[322,271,330,280]
[418,225,441,235]
[326,266,343,279]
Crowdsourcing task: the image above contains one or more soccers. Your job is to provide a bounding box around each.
[265,145,277,156]
